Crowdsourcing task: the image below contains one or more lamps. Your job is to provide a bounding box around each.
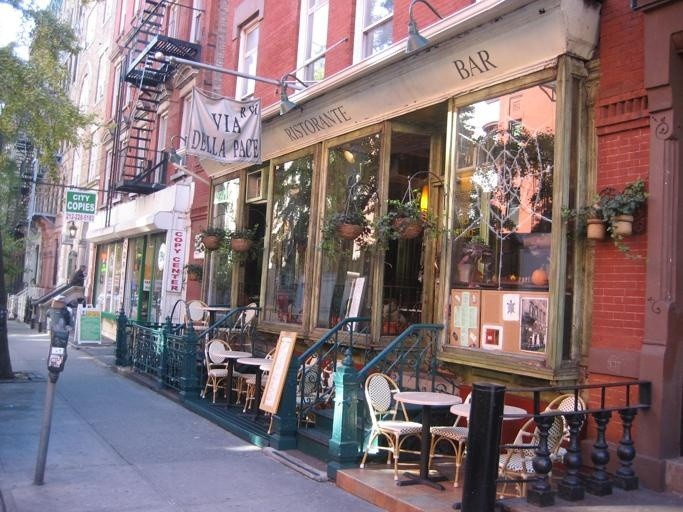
[279,74,308,116]
[405,0,443,54]
[169,134,187,164]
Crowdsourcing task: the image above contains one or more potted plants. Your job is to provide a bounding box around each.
[602,174,651,260]
[321,212,375,260]
[560,178,616,241]
[194,225,261,251]
[375,198,446,252]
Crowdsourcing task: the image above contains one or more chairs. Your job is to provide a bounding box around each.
[202,339,343,436]
[184,300,257,352]
[359,373,423,480]
[506,394,587,479]
[494,411,564,500]
[427,391,473,488]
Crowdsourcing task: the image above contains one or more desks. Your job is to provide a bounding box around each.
[450,405,527,420]
